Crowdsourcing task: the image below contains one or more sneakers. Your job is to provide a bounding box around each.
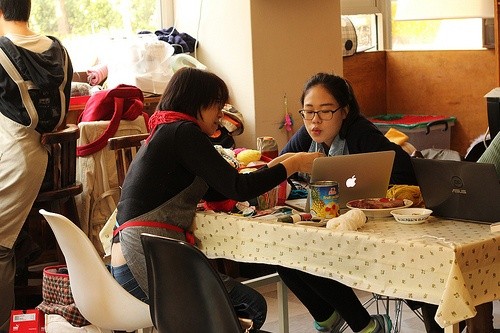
[312,313,348,333]
[369,313,392,333]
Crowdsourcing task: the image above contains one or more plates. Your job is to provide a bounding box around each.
[346,197,413,218]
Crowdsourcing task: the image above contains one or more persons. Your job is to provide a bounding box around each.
[0,0,74,333]
[111,66,324,333]
[277,72,417,333]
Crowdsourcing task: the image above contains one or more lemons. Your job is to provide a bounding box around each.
[236,149,261,165]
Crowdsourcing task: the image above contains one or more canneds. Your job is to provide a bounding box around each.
[309,180,340,221]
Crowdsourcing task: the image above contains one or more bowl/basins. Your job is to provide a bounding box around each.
[390,208,433,224]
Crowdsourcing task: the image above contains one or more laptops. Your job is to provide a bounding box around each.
[283,151,396,213]
[410,157,500,222]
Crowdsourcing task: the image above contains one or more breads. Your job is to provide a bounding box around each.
[394,186,421,205]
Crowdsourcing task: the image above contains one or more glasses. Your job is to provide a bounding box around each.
[298,106,342,121]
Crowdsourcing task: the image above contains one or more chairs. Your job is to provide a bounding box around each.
[39,208,156,333]
[139,233,245,333]
[15,122,83,307]
[109,133,150,197]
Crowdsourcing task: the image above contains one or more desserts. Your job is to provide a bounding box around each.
[348,197,404,209]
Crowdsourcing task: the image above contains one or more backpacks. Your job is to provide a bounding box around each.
[75,83,145,156]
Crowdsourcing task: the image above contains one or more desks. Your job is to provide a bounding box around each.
[65,95,162,124]
[185,181,499,333]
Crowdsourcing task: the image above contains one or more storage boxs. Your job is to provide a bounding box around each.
[363,112,456,152]
[9,309,45,333]
